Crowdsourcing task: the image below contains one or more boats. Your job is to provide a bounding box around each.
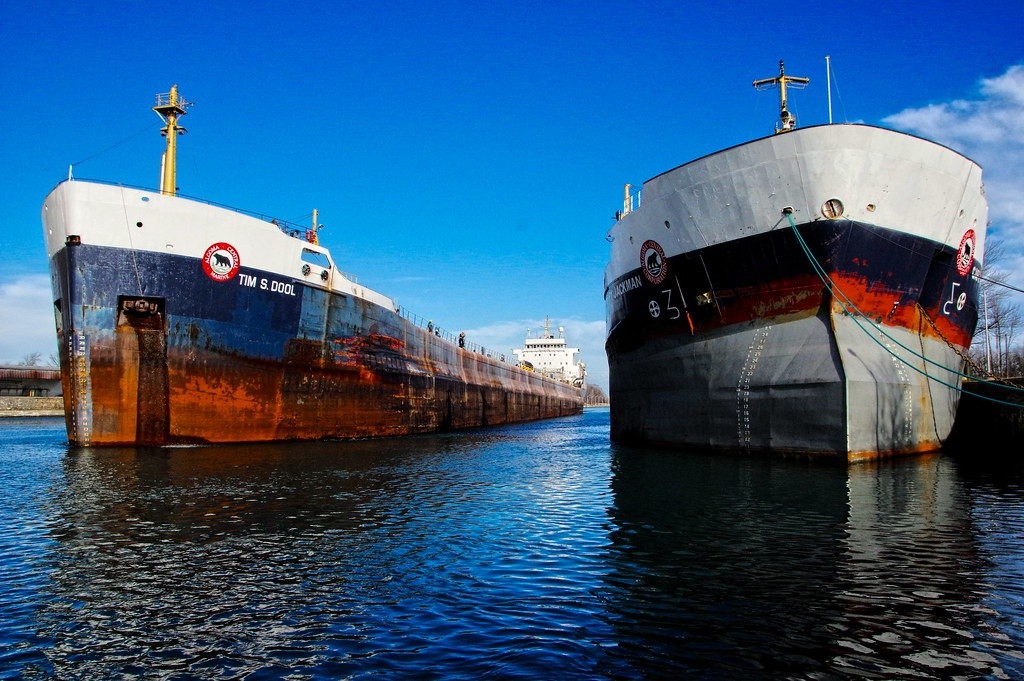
[41,85,586,449]
[603,54,989,461]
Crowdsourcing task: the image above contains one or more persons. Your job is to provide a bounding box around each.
[500,354,505,362]
[427,320,433,333]
[459,331,465,348]
[435,325,441,337]
